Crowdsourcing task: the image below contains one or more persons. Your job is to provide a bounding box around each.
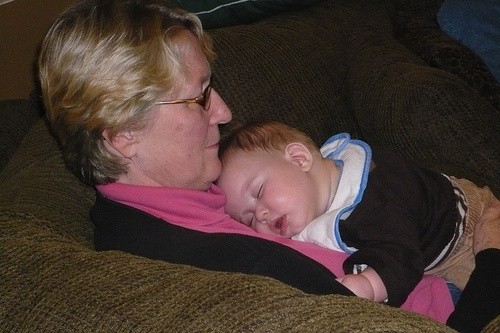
[212,118,498,304]
[38,1,500,332]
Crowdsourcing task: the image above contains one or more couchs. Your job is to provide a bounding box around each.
[0,4,500,333]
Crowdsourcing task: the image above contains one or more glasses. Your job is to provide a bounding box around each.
[155,81,214,111]
